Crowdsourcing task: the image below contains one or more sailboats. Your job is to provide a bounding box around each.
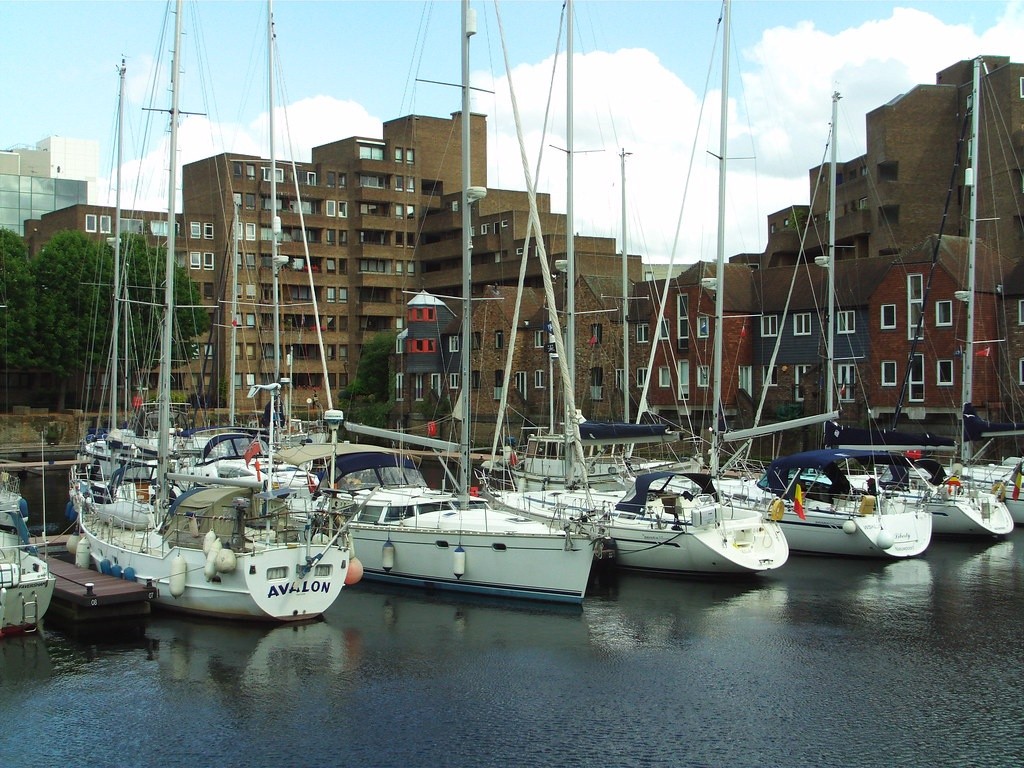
[0,1,1024,638]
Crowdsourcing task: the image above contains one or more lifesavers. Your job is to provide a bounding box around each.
[992,483,1005,501]
[771,500,785,521]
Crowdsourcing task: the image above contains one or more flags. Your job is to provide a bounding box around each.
[1010,462,1022,502]
[794,484,807,520]
[244,441,261,469]
[947,477,961,487]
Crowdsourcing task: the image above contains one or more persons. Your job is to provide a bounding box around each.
[312,389,321,410]
[867,478,876,496]
